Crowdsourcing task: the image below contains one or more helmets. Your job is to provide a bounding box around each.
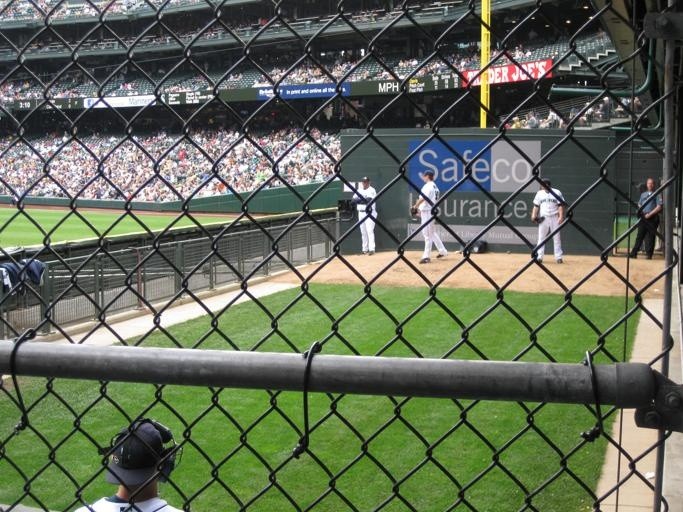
[361,176,370,183]
[424,170,435,175]
[541,179,552,185]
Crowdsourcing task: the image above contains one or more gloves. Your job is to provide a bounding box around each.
[535,216,545,224]
[411,208,418,216]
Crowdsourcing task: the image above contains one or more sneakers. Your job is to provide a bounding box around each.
[557,259,563,264]
[419,254,448,264]
[362,250,374,256]
[537,259,542,263]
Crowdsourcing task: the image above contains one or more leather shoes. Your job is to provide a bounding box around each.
[625,253,637,258]
[645,254,652,259]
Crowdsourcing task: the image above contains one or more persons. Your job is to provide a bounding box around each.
[74,420,185,512]
[414,170,447,263]
[626,179,661,259]
[530,178,565,263]
[352,177,377,256]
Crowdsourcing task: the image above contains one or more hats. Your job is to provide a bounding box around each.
[105,422,163,486]
